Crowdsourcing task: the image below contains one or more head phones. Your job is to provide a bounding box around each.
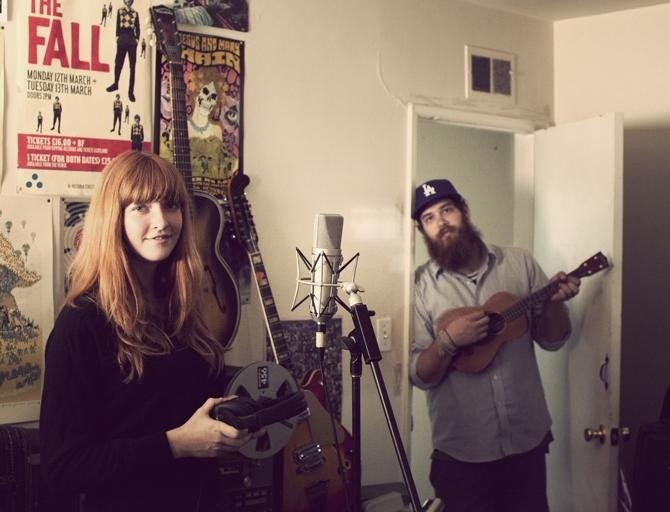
[209,389,306,435]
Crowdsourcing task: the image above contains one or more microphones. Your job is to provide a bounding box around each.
[309,212,344,348]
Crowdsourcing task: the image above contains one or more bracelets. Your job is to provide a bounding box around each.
[438,329,456,358]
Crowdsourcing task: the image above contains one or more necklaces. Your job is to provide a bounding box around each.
[188,118,209,134]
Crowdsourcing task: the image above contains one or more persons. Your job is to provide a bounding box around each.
[36,0,146,151]
[170,67,228,171]
[40,150,252,512]
[409,180,582,512]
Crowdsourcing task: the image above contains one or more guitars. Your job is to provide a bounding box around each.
[146,3,240,350]
[225,174,362,512]
[437,251,610,372]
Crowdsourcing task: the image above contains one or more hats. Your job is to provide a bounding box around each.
[413,179,460,218]
